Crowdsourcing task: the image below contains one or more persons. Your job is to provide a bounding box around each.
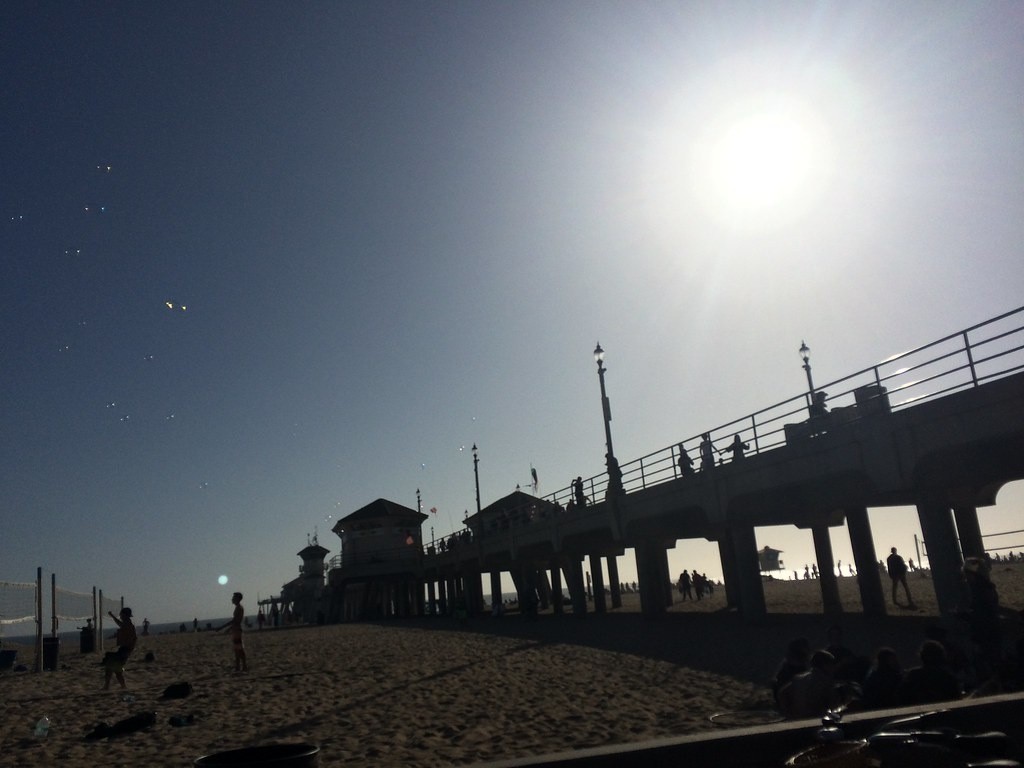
[256,610,266,629]
[679,569,694,602]
[811,391,830,417]
[271,609,279,626]
[143,618,149,633]
[692,570,714,599]
[949,555,1007,694]
[887,548,915,605]
[216,593,249,672]
[439,529,471,552]
[767,638,963,721]
[193,618,198,633]
[678,448,696,476]
[698,435,722,471]
[283,607,290,624]
[86,618,93,629]
[100,608,137,690]
[567,499,577,513]
[571,477,584,506]
[724,435,749,460]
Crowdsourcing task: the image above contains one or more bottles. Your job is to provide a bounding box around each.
[34,715,51,741]
[128,689,135,705]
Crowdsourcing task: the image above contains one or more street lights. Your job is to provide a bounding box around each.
[471,442,481,512]
[415,488,422,512]
[594,342,615,457]
[799,340,814,403]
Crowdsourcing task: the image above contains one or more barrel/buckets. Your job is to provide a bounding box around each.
[193,743,322,768]
[0,650,17,668]
[80,630,96,653]
[43,637,59,671]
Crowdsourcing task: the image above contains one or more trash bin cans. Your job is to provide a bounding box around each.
[0,648,17,678]
[192,743,320,768]
[42,636,61,672]
